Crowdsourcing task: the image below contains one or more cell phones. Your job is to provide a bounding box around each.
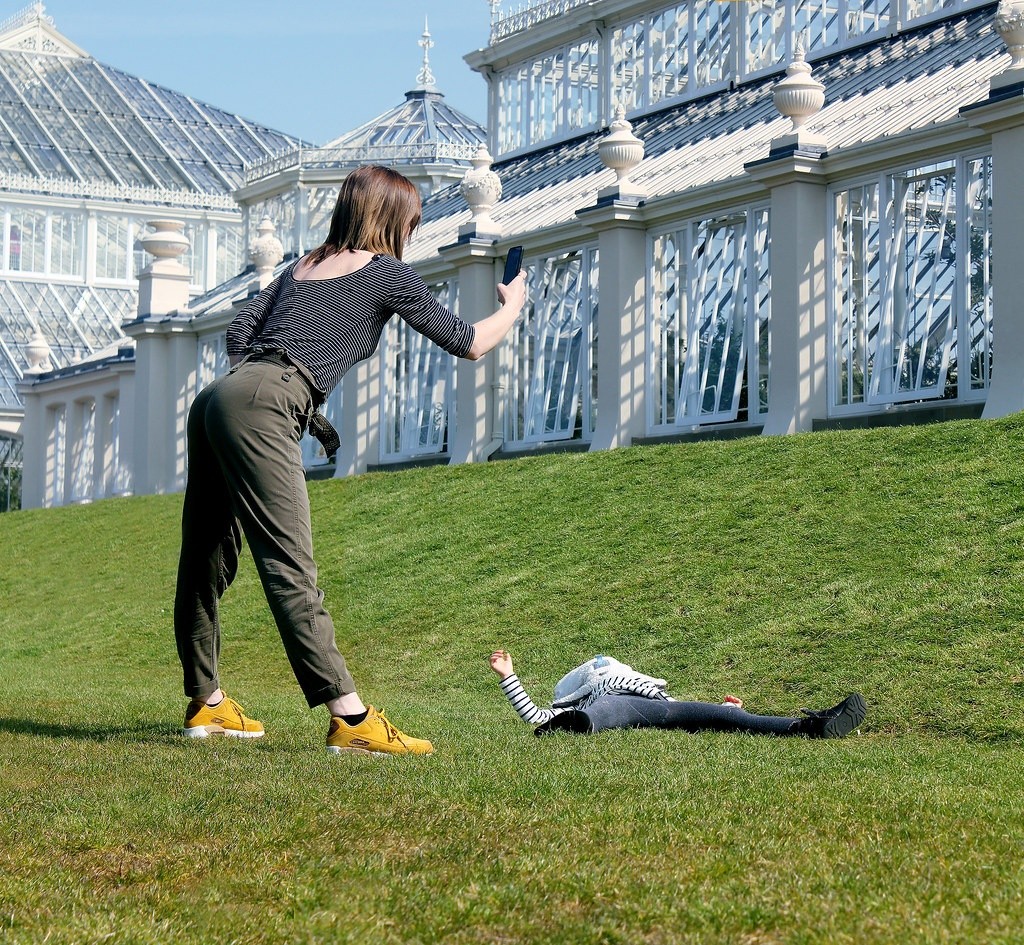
[502,246,524,286]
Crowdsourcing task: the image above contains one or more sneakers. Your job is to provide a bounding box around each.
[326,704,434,759]
[183,690,266,741]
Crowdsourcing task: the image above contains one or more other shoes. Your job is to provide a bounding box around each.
[533,710,590,738]
[794,692,867,739]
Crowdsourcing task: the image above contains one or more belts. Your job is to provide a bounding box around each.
[245,348,341,459]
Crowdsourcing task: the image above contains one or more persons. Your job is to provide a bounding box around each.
[489,651,866,738]
[173,167,527,757]
